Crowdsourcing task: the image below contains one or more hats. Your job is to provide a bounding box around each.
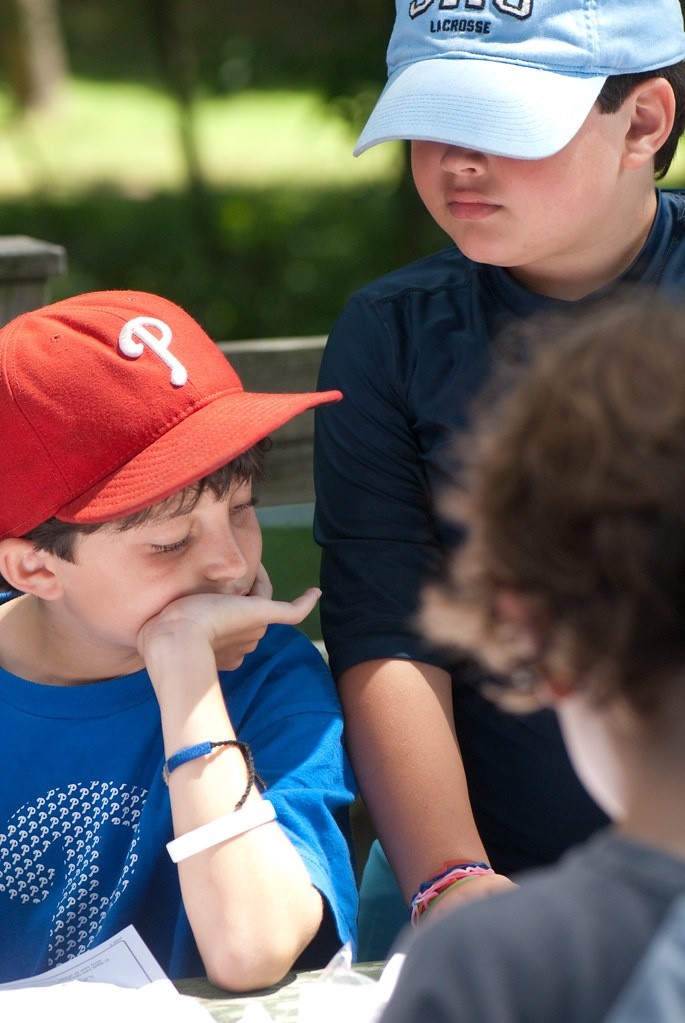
[0,291,343,540]
[352,0,685,160]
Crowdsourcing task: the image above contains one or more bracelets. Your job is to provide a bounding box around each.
[408,859,496,929]
[162,741,268,809]
[167,801,278,865]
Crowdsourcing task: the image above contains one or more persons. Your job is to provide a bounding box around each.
[312,2,684,965]
[0,289,364,991]
[381,295,685,1023]
